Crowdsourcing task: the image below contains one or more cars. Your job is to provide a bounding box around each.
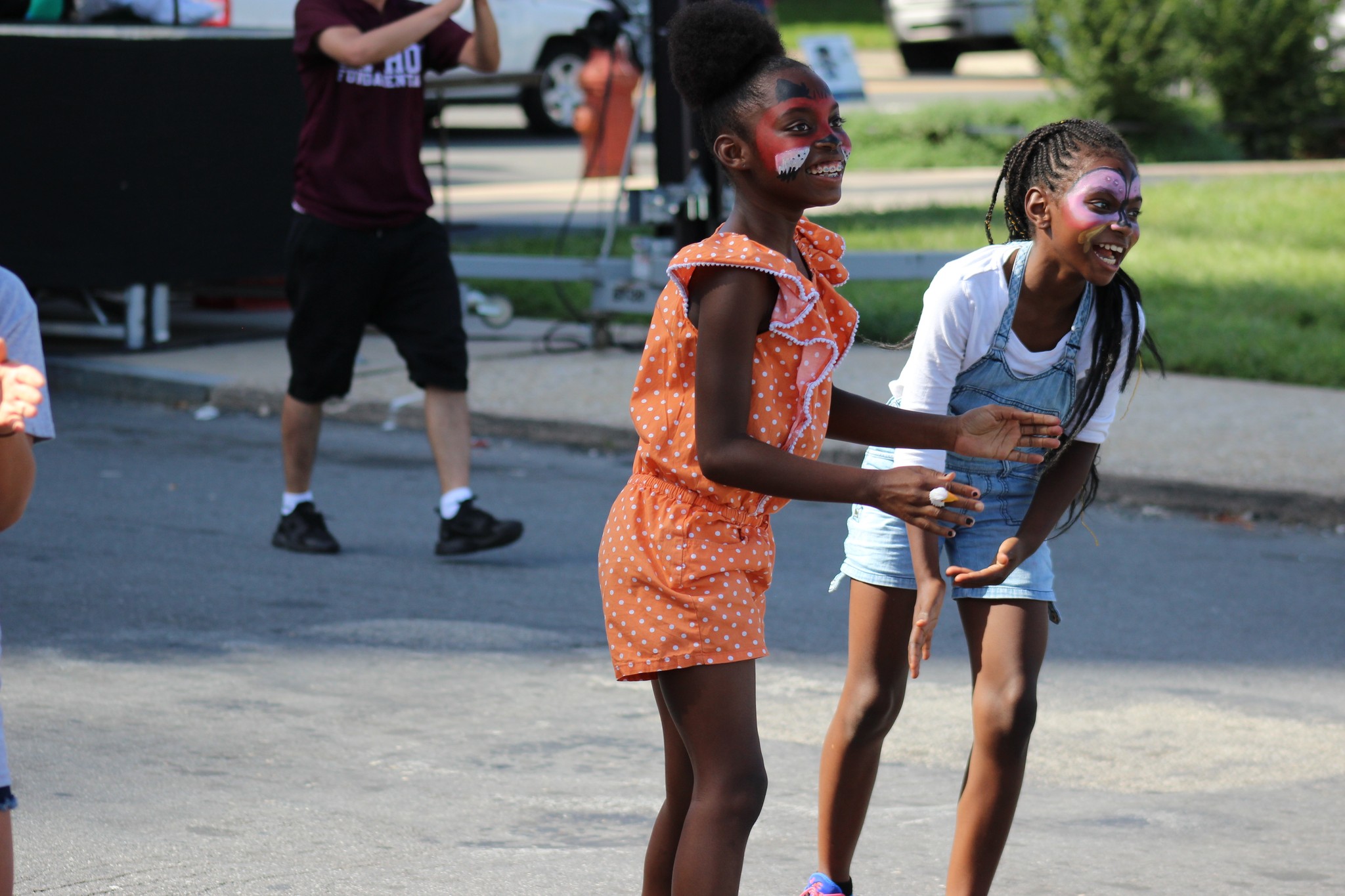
[886,0,1043,75]
[22,1,627,146]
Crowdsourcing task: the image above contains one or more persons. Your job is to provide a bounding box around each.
[798,121,1169,896]
[571,0,790,230]
[0,259,57,896]
[595,0,1066,896]
[272,0,525,558]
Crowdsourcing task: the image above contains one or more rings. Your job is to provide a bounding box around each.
[928,486,961,508]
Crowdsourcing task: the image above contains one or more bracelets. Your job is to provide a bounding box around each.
[0,430,18,438]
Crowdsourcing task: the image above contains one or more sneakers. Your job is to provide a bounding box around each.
[435,494,523,554]
[270,502,337,551]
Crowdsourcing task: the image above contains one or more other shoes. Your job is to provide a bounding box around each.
[799,871,854,896]
